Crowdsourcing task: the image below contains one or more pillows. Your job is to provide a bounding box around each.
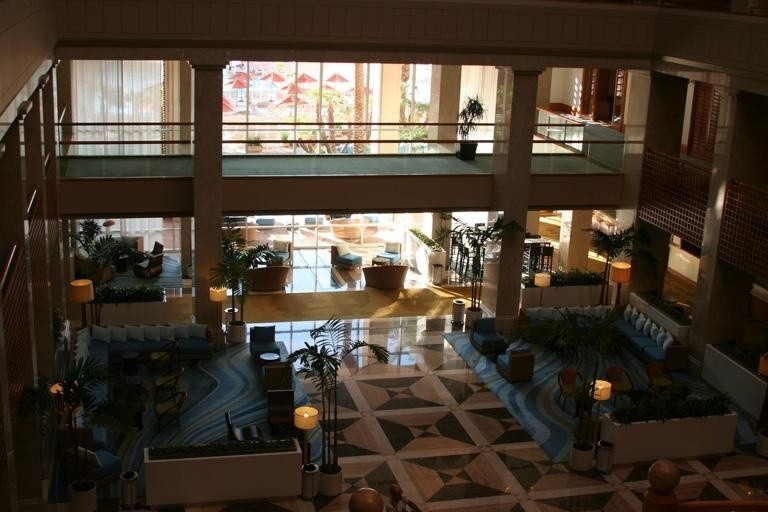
[521,304,682,355]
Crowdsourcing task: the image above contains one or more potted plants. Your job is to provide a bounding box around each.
[11,209,391,512]
[520,267,608,311]
[572,390,739,469]
[445,211,504,328]
[560,308,634,471]
[458,95,485,160]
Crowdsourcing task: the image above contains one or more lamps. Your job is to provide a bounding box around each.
[588,381,612,452]
[610,261,632,306]
[532,273,556,304]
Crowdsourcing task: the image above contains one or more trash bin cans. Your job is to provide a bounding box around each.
[433,264,442,285]
[453,299,465,328]
[300,463,320,501]
[597,441,615,474]
[225,308,238,335]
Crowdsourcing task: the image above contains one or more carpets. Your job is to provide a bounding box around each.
[439,330,758,461]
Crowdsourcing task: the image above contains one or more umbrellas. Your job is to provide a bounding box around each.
[221,69,374,122]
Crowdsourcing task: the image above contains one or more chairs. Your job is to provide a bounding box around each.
[249,216,411,289]
[471,317,536,382]
[524,227,555,272]
[557,362,673,408]
